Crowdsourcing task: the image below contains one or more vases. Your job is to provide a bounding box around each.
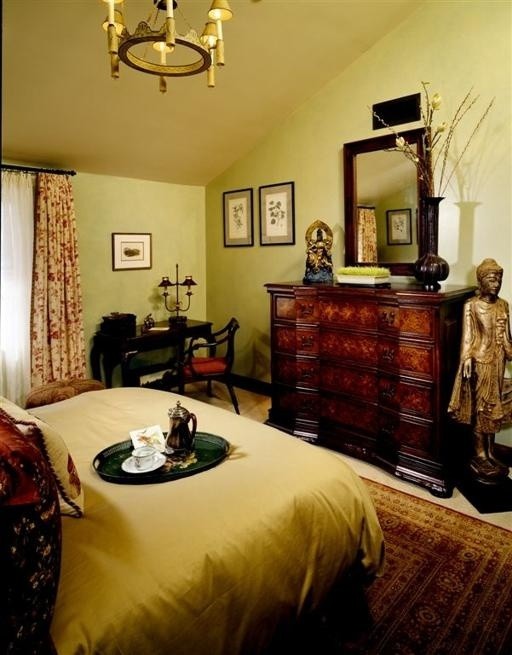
[413,197,450,292]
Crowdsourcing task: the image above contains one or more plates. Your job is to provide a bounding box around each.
[121,454,167,474]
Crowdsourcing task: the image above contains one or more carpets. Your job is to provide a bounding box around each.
[345,475,512,655]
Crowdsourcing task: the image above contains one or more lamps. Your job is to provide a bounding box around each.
[104,1,233,93]
[158,263,198,325]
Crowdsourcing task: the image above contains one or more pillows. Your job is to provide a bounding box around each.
[0,410,61,655]
[0,396,84,519]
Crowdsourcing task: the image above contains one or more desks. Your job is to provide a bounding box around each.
[91,312,216,397]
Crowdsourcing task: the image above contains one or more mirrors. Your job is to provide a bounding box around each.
[354,142,418,263]
[344,127,431,275]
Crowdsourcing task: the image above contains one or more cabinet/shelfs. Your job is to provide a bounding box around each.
[263,282,479,499]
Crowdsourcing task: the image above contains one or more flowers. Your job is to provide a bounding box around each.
[366,81,496,197]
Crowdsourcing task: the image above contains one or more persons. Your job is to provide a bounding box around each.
[308,229,332,268]
[448,257,512,478]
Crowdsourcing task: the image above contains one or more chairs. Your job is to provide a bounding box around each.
[175,317,240,416]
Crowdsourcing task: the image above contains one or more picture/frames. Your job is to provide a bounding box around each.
[223,188,253,247]
[385,209,412,245]
[112,232,152,271]
[259,182,295,246]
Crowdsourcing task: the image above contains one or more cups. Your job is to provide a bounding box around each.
[132,446,156,471]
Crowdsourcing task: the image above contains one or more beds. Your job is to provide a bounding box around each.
[0,387,385,654]
